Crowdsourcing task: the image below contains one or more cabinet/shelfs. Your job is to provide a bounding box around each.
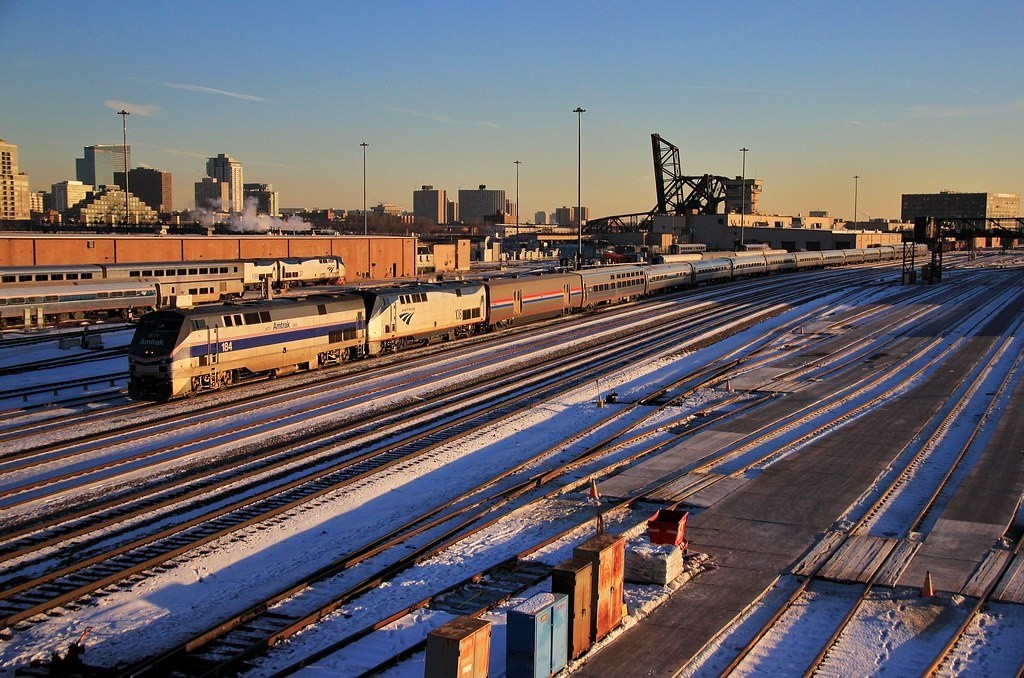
[423,533,627,678]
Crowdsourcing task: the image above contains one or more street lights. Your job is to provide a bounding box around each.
[514,161,522,244]
[360,142,370,236]
[739,147,749,245]
[118,110,130,225]
[853,175,860,230]
[572,107,586,269]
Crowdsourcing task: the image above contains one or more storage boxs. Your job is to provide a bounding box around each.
[647,509,689,546]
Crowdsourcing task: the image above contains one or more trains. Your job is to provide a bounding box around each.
[0,279,164,329]
[0,255,347,292]
[125,244,929,405]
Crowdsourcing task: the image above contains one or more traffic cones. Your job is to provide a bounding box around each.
[919,570,934,596]
